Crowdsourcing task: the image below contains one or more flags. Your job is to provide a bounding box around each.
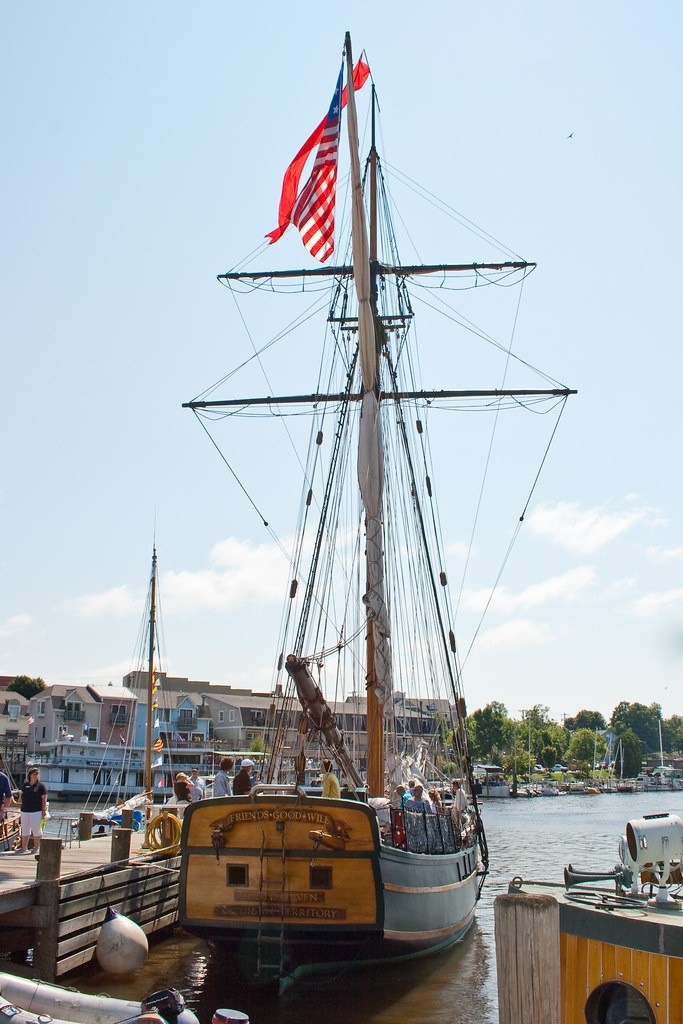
[28,715,34,724]
[176,733,182,741]
[285,57,345,264]
[82,722,88,733]
[152,666,165,788]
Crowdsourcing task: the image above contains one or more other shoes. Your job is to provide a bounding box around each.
[33,847,38,854]
[17,849,30,854]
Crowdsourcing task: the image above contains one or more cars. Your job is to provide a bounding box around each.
[588,760,608,771]
[533,765,546,774]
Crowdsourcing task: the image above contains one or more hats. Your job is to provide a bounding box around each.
[191,766,199,772]
[241,759,255,767]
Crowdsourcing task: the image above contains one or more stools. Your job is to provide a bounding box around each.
[58,817,81,849]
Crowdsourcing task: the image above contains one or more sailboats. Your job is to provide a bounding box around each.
[70,523,176,841]
[177,29,580,975]
[634,719,683,791]
[0,751,21,852]
[591,727,644,792]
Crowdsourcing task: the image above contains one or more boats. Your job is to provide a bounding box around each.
[473,763,511,799]
[508,782,600,797]
[539,781,559,796]
[644,772,672,790]
[506,813,683,1024]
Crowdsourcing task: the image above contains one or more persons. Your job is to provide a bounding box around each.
[15,768,48,855]
[0,772,12,838]
[311,760,468,830]
[174,756,261,819]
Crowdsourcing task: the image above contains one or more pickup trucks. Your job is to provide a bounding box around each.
[546,764,568,773]
